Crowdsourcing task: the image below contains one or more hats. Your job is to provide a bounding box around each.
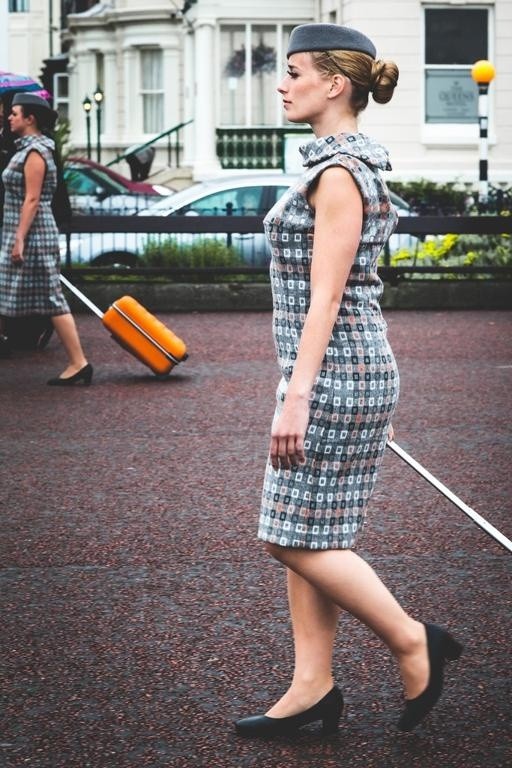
[286,22,377,59]
[10,93,51,109]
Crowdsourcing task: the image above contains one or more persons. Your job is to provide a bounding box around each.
[25,124,74,353]
[1,89,93,393]
[235,22,463,740]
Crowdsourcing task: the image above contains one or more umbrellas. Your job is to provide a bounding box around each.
[1,69,48,100]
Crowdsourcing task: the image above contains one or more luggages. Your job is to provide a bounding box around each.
[58,272,189,384]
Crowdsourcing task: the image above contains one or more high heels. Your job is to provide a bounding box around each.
[45,363,93,387]
[396,621,463,730]
[234,684,344,738]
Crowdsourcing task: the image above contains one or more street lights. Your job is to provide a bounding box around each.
[82,94,94,162]
[468,56,497,208]
[93,83,105,165]
